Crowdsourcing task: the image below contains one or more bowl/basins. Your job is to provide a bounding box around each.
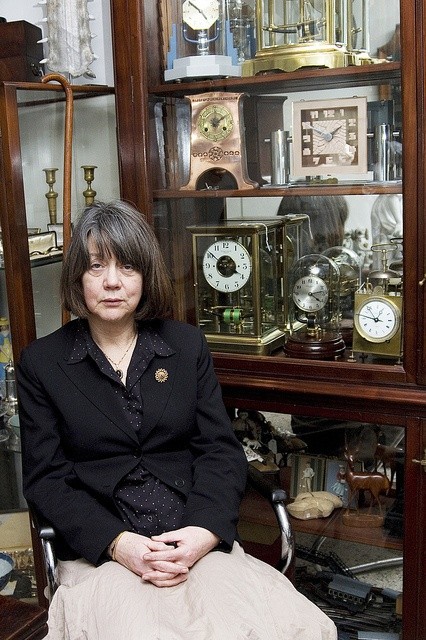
[81,324,139,380]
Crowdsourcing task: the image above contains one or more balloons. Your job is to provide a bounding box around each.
[202,239,250,293]
[292,274,329,313]
[300,105,359,167]
[354,296,401,344]
[196,104,233,142]
[181,0,219,30]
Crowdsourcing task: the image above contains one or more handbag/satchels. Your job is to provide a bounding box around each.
[109,531,127,563]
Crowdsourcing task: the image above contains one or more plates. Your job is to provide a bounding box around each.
[214,372,424,638]
[0,77,132,640]
[111,0,426,389]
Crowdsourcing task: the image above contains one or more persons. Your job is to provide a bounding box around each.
[13,200,340,639]
[277,196,348,258]
